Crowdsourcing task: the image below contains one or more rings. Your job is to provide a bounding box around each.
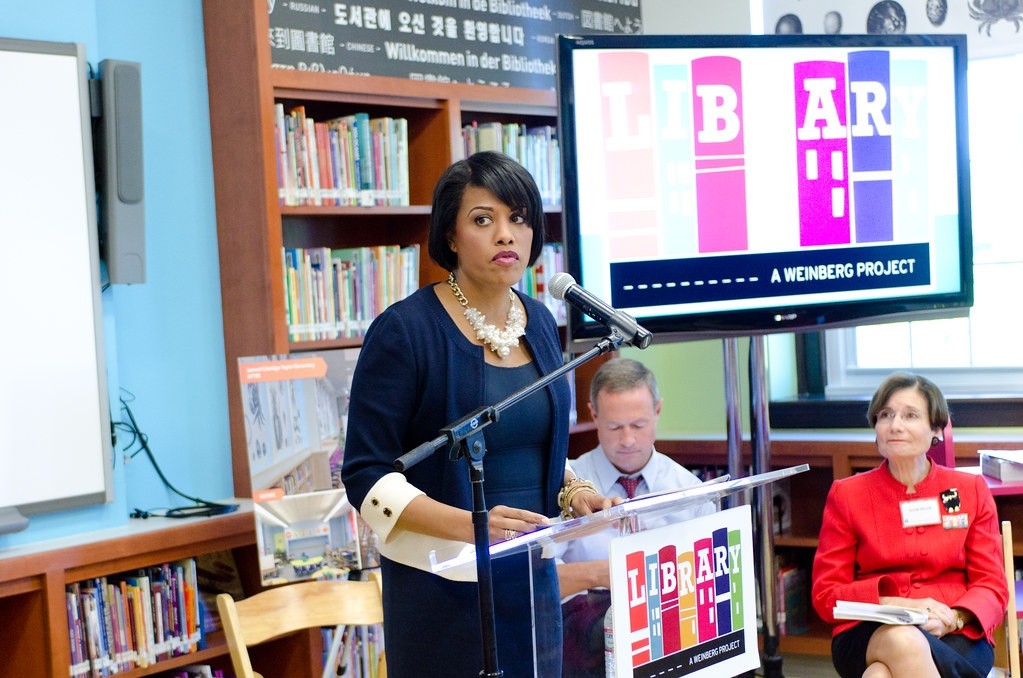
[926,607,932,613]
[504,530,517,540]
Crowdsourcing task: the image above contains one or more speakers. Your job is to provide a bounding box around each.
[98,58,147,286]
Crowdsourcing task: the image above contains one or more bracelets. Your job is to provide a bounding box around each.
[558,477,599,520]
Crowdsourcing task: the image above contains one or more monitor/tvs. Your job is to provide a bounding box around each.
[554,31,974,353]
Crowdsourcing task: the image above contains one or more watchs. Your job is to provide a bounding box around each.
[954,608,964,631]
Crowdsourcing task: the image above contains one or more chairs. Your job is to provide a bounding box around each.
[994,518,1023,678]
[215,566,385,678]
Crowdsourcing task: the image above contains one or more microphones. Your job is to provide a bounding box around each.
[548,272,654,350]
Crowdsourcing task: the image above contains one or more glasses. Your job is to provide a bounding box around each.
[873,408,930,425]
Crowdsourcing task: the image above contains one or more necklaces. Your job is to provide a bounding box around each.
[448,272,527,360]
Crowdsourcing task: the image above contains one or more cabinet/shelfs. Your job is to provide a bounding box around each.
[0,505,325,678]
[656,428,1023,656]
[200,0,620,678]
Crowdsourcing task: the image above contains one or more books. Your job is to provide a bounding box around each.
[278,243,420,342]
[321,623,386,678]
[275,103,410,207]
[66,550,246,678]
[513,243,566,321]
[461,119,562,206]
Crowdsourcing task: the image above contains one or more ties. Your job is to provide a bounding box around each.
[618,475,645,532]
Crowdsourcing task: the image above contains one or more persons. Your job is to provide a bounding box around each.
[812,374,1010,678]
[342,150,625,678]
[552,358,716,661]
